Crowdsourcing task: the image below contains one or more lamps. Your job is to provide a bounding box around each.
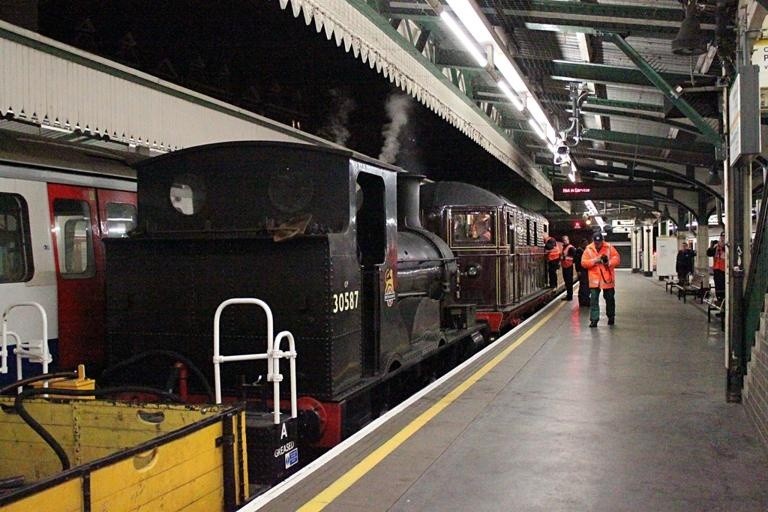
[669,1,742,85]
[425,0,607,231]
[702,156,725,187]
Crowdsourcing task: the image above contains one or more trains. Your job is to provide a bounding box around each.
[0,138,564,511]
[0,172,138,399]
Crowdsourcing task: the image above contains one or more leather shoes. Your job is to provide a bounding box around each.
[589,321,597,327]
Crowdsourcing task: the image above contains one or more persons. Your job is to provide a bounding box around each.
[579,231,620,327]
[706,230,724,317]
[677,241,696,280]
[575,237,591,307]
[560,235,576,301]
[543,232,560,288]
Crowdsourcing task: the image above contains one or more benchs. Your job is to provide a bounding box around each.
[664,272,727,333]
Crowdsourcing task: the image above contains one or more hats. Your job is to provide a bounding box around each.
[592,232,603,240]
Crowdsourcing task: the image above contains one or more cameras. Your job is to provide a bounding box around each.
[601,254,608,263]
[711,240,718,244]
